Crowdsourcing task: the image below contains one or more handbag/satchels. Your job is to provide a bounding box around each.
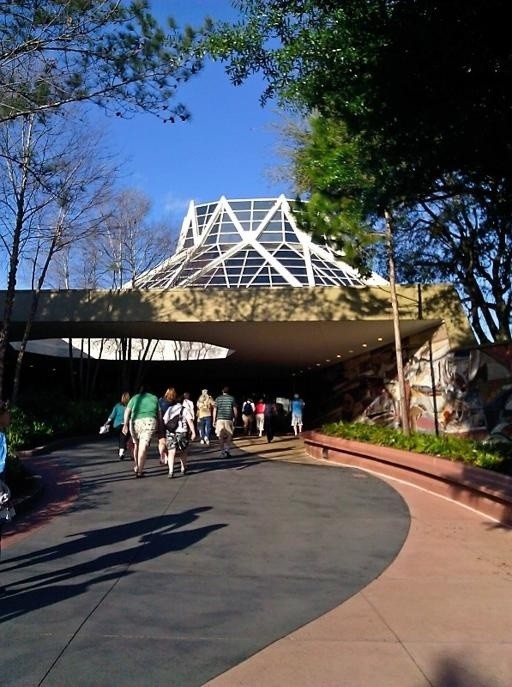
[165,414,180,432]
[99,424,110,434]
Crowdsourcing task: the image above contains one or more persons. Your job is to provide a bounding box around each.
[104,378,238,479]
[240,391,307,443]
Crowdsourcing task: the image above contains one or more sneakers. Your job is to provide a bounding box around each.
[119,450,232,479]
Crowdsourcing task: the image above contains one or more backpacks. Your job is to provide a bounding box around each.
[244,401,253,415]
